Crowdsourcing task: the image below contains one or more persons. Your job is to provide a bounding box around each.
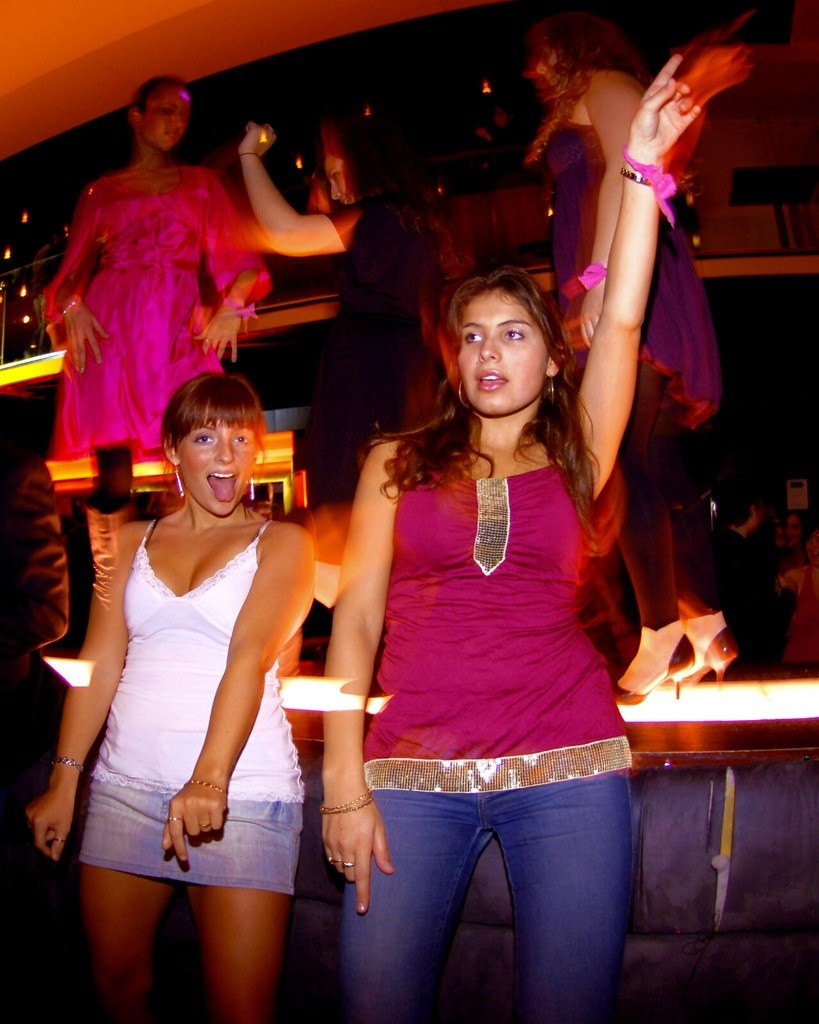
[0,392,66,1024]
[44,77,273,609]
[524,10,737,705]
[322,53,701,1024]
[27,370,311,1024]
[239,89,479,660]
[693,473,819,682]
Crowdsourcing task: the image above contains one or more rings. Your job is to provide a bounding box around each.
[170,817,184,822]
[200,823,211,828]
[53,837,66,842]
[204,337,212,344]
[329,856,344,862]
[342,862,355,868]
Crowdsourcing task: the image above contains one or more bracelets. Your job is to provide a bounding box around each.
[185,780,227,797]
[62,298,84,315]
[320,790,374,815]
[241,152,257,156]
[52,758,84,772]
[621,168,654,187]
[222,301,239,308]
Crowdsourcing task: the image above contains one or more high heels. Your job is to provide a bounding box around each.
[661,625,738,686]
[612,634,695,707]
